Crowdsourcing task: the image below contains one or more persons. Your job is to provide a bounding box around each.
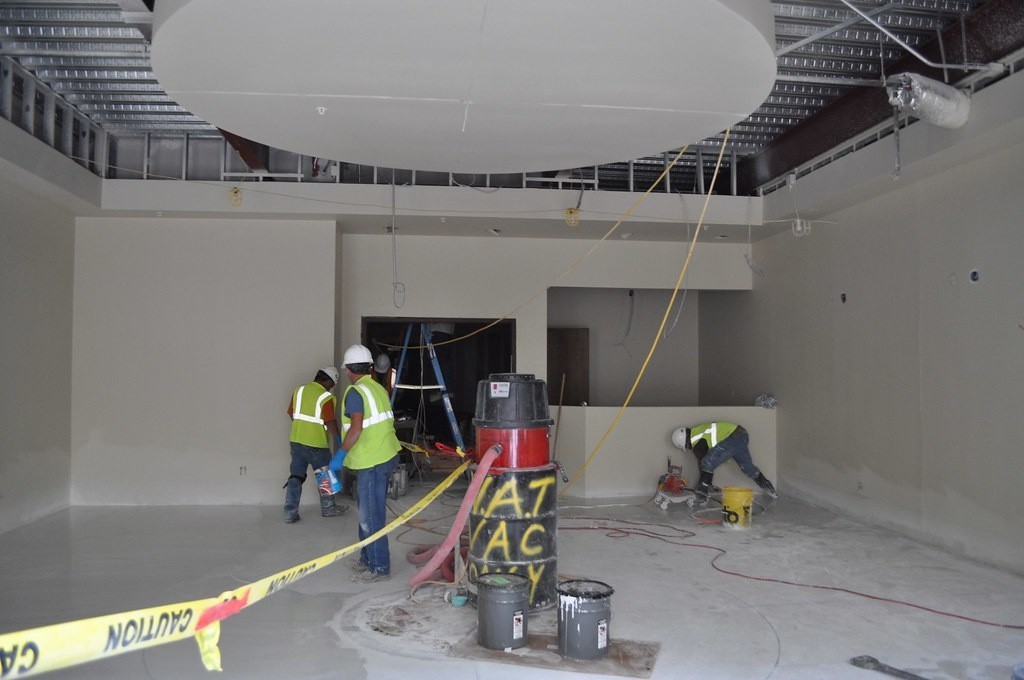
[371,354,397,399]
[284,366,343,524]
[328,344,402,583]
[673,421,778,503]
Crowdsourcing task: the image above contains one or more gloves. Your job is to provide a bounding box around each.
[334,435,343,448]
[327,447,348,471]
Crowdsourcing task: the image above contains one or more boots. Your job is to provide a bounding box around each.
[695,471,713,503]
[754,472,776,495]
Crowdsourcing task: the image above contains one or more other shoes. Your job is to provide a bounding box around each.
[286,513,300,524]
[322,503,349,517]
[352,560,368,570]
[350,568,391,583]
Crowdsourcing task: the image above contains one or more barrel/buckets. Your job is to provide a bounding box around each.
[314,465,343,497]
[720,487,754,533]
[465,460,560,615]
[472,372,556,467]
[554,577,615,661]
[472,572,531,650]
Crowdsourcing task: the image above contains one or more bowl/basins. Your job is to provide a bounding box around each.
[449,596,468,608]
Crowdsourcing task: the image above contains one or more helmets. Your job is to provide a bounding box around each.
[320,366,339,385]
[374,352,391,374]
[671,427,686,452]
[341,344,375,370]
